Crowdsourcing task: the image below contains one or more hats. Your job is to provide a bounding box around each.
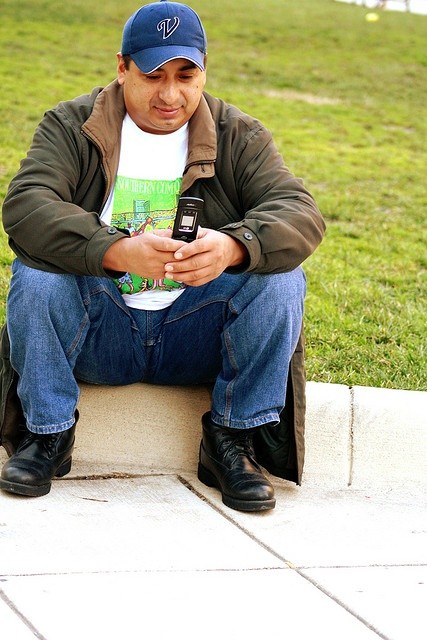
[122,0,206,73]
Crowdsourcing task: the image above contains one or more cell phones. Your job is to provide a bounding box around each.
[170,196,203,243]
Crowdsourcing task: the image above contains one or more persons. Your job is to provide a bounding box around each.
[0,1,327,512]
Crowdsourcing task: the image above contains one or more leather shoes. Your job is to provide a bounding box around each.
[1,409,80,497]
[198,412,275,512]
